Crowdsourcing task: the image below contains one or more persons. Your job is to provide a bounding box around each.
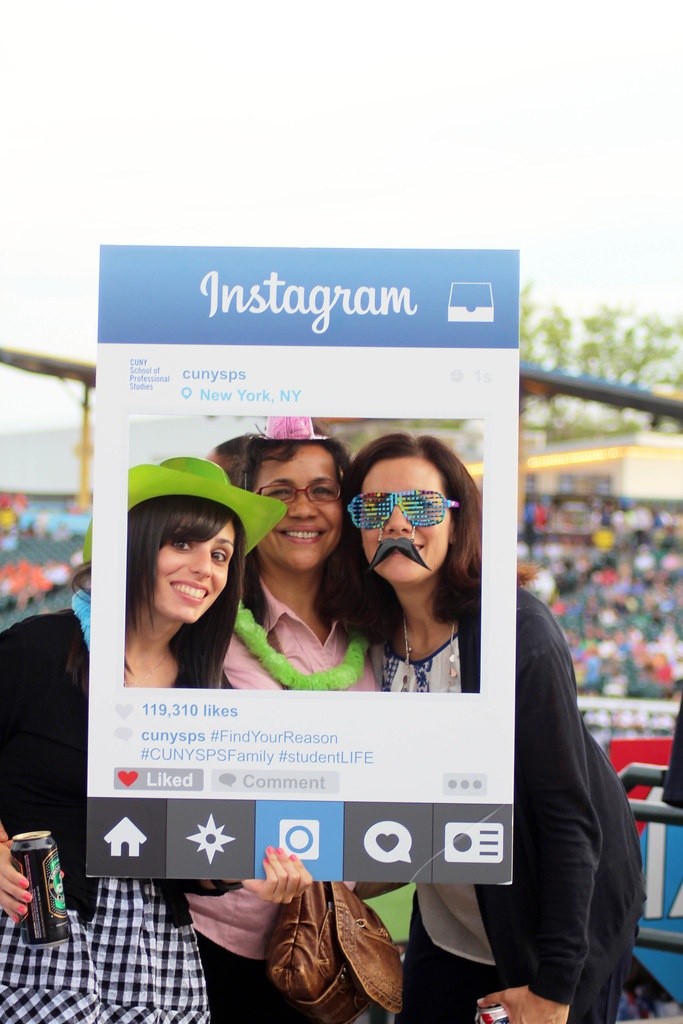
[0,458,312,1024]
[184,418,409,1024]
[515,492,683,700]
[1,492,83,610]
[340,434,647,1024]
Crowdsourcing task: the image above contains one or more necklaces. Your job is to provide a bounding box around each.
[400,613,457,693]
[70,587,90,653]
[231,600,369,692]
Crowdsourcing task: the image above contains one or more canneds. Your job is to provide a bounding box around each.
[474,1003,509,1024]
[9,830,70,950]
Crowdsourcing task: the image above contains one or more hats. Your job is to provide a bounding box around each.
[78,456,289,568]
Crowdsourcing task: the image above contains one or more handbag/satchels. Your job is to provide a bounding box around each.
[266,882,404,1024]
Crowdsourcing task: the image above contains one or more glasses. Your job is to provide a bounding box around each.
[257,480,346,503]
[345,488,460,527]
